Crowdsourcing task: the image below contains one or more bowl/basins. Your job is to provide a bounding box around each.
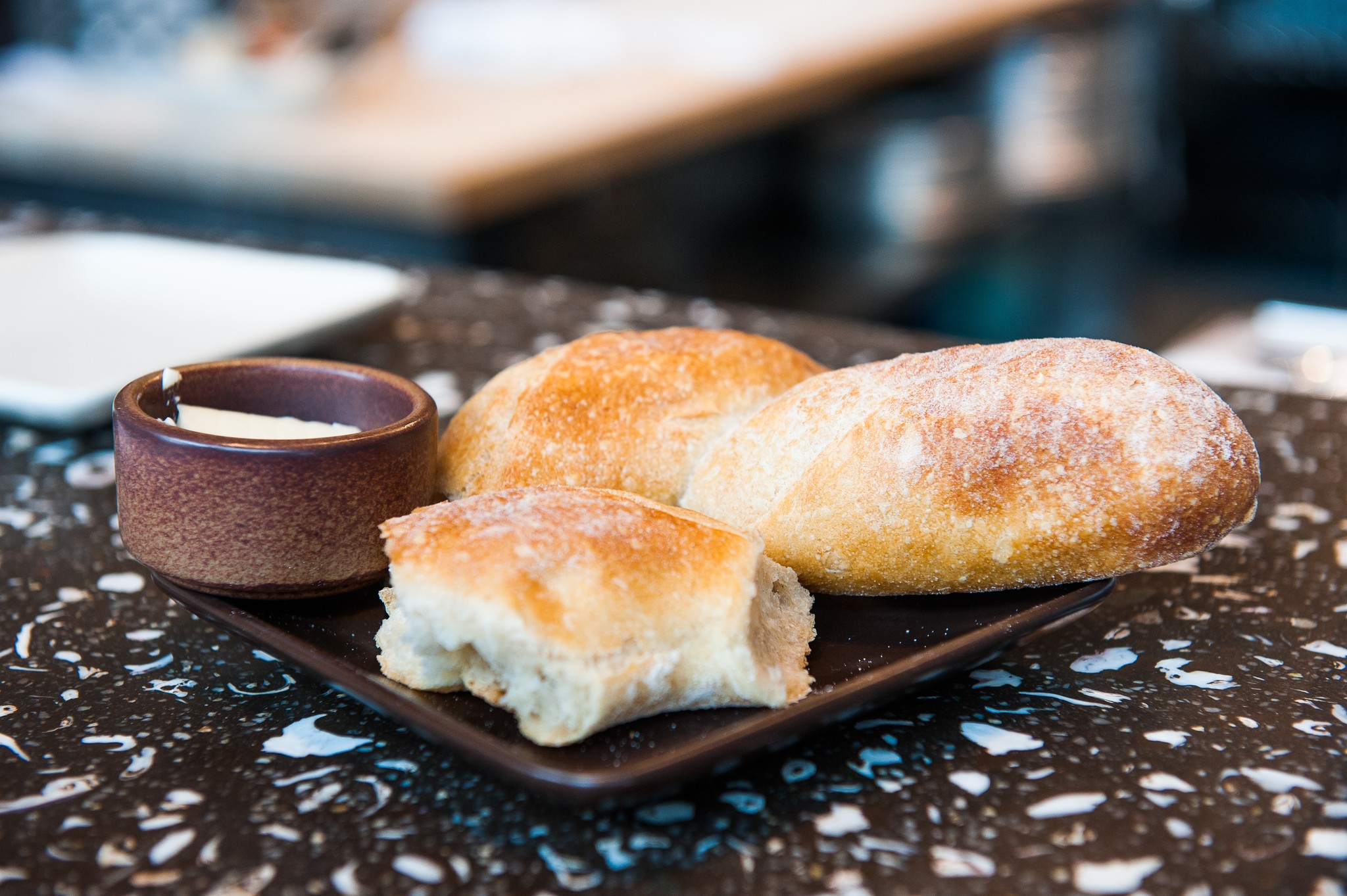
[113,357,439,600]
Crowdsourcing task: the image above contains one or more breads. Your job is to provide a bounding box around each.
[680,337,1262,597]
[374,484,818,746]
[434,326,827,505]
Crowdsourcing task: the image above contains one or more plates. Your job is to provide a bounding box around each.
[152,414,1118,806]
[0,231,407,430]
